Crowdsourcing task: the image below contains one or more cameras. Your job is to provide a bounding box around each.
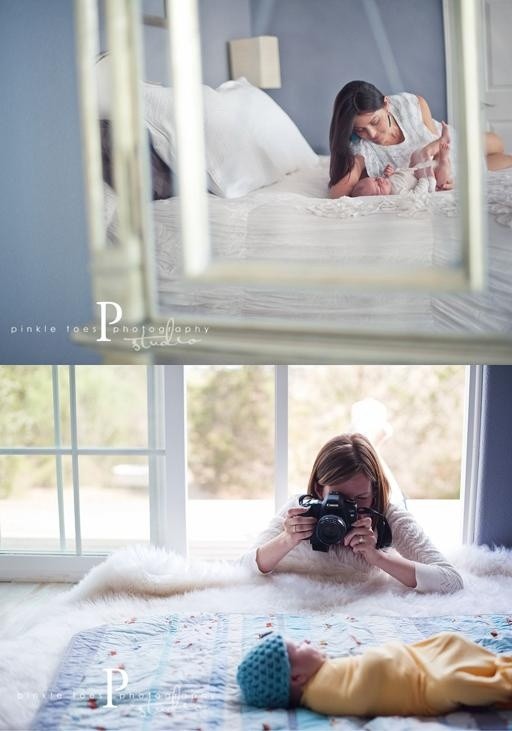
[298,493,358,552]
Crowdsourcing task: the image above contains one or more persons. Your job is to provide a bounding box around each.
[351,120,450,197]
[236,629,512,716]
[239,433,464,595]
[328,80,512,199]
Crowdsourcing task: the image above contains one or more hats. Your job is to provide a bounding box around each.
[236,630,293,711]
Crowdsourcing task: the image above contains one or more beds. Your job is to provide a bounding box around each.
[101,156,512,315]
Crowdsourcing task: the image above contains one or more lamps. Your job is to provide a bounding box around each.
[231,36,280,88]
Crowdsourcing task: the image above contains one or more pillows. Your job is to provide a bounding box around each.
[89,78,319,203]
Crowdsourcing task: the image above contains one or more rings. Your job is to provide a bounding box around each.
[359,536,363,544]
[293,525,296,533]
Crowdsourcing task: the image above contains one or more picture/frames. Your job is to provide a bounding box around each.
[143,0,166,28]
